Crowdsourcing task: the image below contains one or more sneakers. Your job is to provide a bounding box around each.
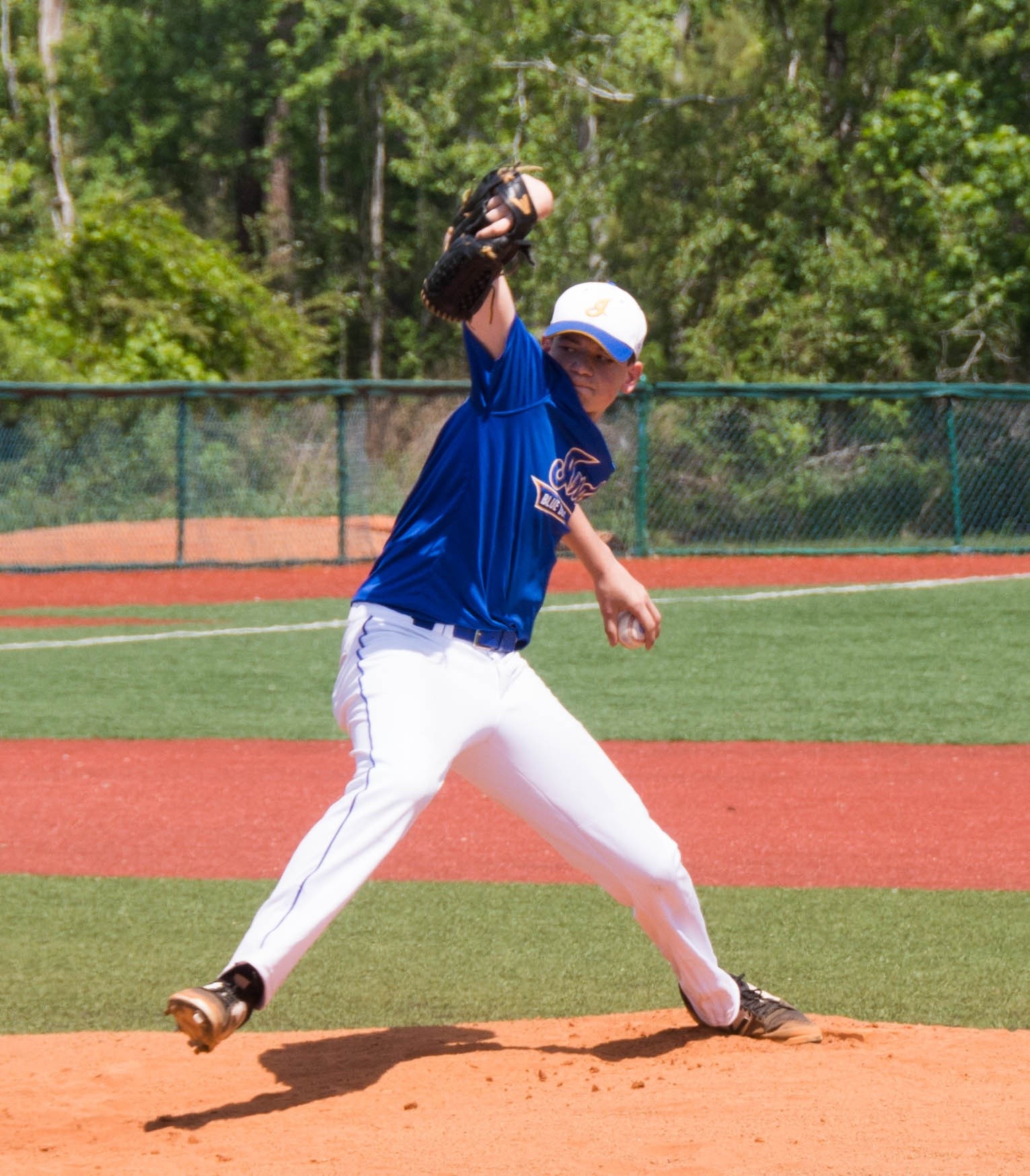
[164,983,246,1054]
[678,974,823,1045]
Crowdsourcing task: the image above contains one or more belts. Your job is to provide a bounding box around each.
[412,617,516,652]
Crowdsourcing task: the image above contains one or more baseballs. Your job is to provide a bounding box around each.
[616,610,647,650]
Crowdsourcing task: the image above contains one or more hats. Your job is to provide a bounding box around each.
[542,281,647,362]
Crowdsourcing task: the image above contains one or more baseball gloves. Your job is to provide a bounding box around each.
[415,158,543,330]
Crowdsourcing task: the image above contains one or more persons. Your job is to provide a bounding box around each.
[163,163,826,1055]
